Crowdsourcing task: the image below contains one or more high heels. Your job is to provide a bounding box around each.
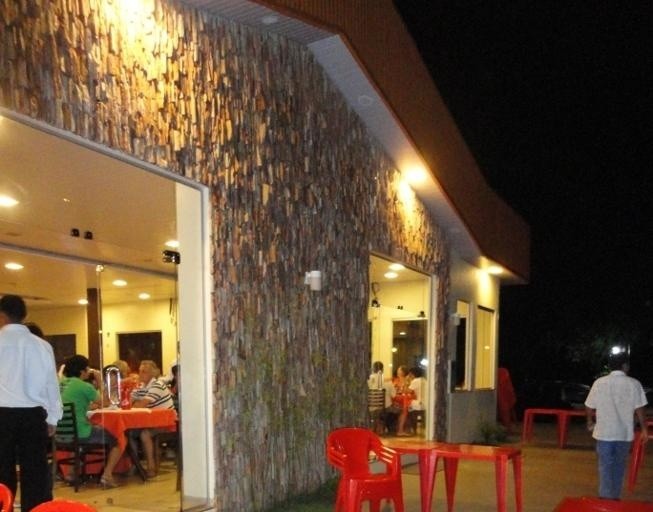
[100,475,120,490]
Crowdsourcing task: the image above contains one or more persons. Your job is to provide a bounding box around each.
[585,352,646,499]
[56,354,179,486]
[0,295,64,510]
[372,361,430,436]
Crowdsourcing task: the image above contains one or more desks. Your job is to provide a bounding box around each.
[369,436,450,512]
[425,443,523,512]
[522,408,653,511]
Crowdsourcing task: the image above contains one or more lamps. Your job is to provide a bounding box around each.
[304,270,323,292]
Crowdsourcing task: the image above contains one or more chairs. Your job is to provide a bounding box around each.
[0,483,14,512]
[408,409,425,437]
[30,499,97,512]
[50,402,109,492]
[367,388,386,434]
[326,427,404,512]
[133,399,179,471]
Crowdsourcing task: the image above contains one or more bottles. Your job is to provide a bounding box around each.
[121,388,130,410]
